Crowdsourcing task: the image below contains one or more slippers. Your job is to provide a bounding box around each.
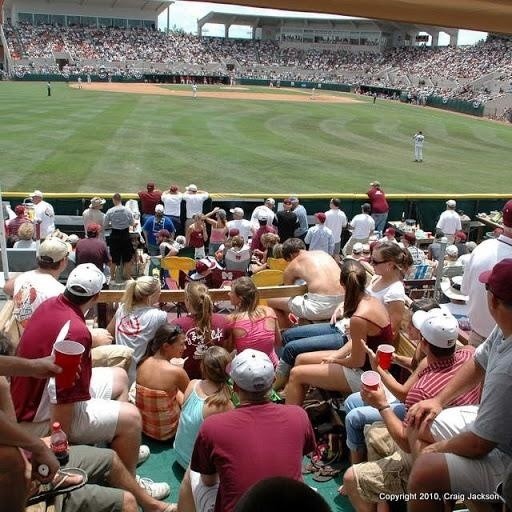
[162,502,178,512]
[28,469,87,506]
[302,459,325,474]
[312,466,341,482]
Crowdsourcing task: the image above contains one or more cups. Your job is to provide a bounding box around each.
[52,341,86,397]
[376,343,395,371]
[169,357,185,375]
[359,370,382,393]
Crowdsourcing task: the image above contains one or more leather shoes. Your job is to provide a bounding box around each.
[312,423,344,434]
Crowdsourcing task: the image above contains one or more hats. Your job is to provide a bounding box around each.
[66,262,106,297]
[412,308,459,349]
[15,180,504,273]
[225,347,276,393]
[439,274,468,301]
[479,257,512,301]
[502,200,512,228]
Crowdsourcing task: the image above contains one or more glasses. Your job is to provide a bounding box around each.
[168,327,179,340]
[493,481,507,504]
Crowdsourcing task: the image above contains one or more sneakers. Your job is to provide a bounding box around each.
[135,473,170,501]
[137,444,151,464]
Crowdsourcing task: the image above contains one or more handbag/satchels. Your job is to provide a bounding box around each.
[310,432,344,466]
[301,399,332,426]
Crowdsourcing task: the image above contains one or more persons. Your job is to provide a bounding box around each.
[0,180,512,512]
[409,129,426,164]
[0,16,511,122]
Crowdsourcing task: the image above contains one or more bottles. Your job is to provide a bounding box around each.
[49,420,68,467]
